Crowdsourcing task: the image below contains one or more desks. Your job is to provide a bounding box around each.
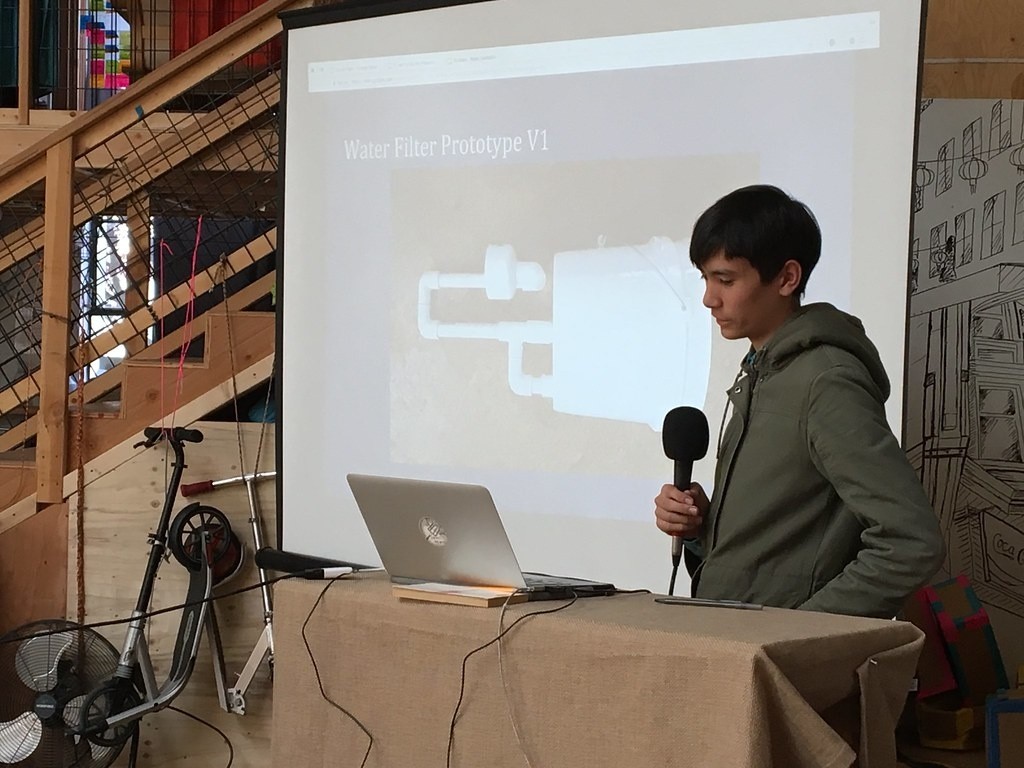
[270,576,926,768]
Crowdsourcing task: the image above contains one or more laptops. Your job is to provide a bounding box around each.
[347,474,616,601]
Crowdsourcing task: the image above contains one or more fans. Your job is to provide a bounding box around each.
[0,619,141,768]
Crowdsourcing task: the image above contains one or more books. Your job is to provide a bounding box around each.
[393,582,530,608]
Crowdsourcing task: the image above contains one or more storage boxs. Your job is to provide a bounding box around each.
[902,574,1024,768]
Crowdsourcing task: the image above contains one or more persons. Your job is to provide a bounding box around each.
[653,184,945,616]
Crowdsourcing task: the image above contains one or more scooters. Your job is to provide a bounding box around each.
[79,424,233,748]
[177,470,275,716]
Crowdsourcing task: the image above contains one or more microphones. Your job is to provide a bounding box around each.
[662,405,710,566]
